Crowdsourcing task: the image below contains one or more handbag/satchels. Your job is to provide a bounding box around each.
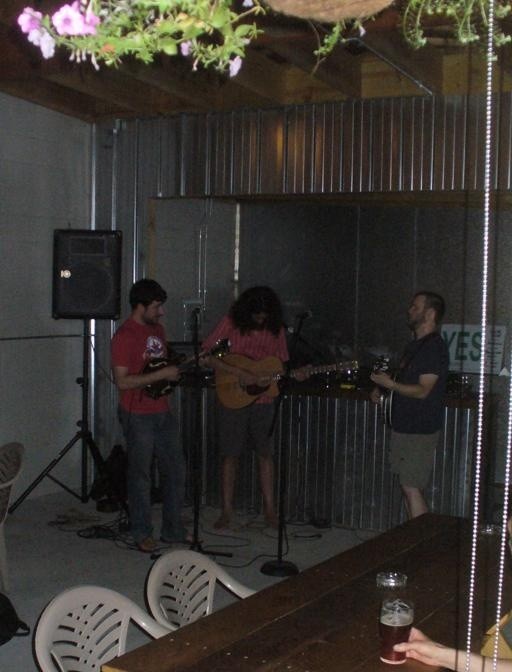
[0,593,29,646]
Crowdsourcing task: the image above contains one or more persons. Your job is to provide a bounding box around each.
[111,279,204,552]
[371,292,450,521]
[203,286,291,527]
[393,626,512,672]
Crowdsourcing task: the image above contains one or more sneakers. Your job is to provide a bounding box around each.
[139,540,159,552]
[161,536,203,544]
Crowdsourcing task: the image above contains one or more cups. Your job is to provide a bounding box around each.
[378,599,414,665]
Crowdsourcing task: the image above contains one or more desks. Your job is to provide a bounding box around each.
[102,512,511,672]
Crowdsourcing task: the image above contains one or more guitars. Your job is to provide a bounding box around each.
[139,337,232,400]
[371,355,403,428]
[215,352,359,410]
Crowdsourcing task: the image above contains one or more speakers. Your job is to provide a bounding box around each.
[51,227,122,321]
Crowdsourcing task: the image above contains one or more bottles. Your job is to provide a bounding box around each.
[375,571,408,641]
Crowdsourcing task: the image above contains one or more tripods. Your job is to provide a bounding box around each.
[8,322,134,523]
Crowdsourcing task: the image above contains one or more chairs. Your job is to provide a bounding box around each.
[33,550,257,671]
[0,441,26,527]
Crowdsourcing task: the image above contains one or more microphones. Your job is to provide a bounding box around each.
[185,307,199,332]
[298,310,312,320]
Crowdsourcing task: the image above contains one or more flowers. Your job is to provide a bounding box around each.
[17,0,268,78]
